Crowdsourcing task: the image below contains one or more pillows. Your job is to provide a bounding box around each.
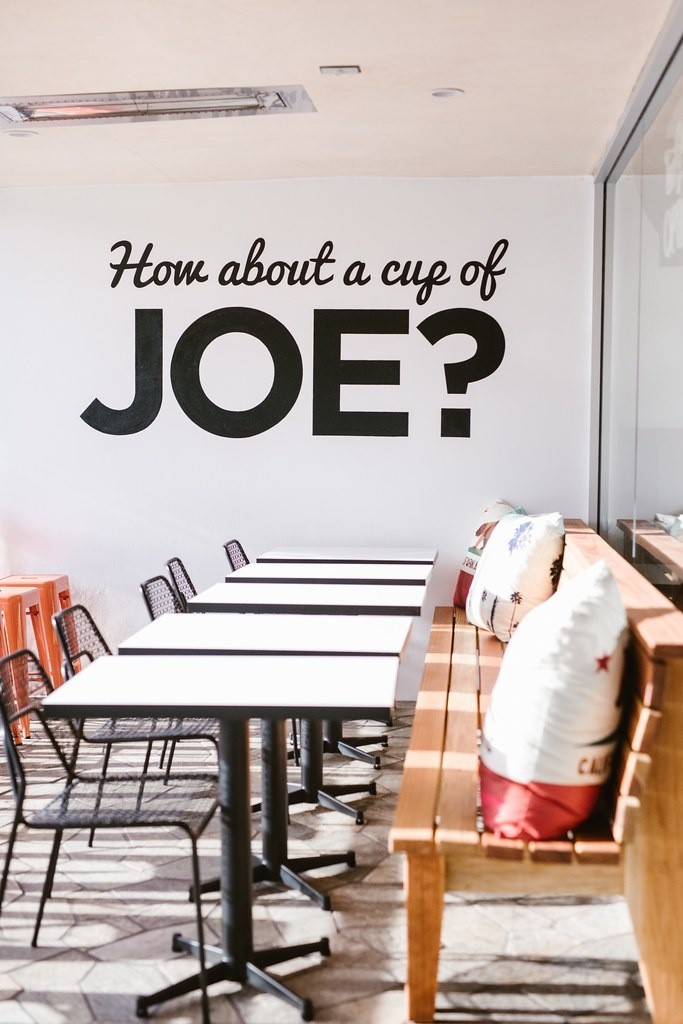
[479,563,629,841]
[465,507,564,643]
[654,514,683,544]
[453,499,516,609]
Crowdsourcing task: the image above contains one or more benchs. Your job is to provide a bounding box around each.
[389,519,683,1024]
[616,518,682,610]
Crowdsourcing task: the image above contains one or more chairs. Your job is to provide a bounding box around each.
[0,539,299,1024]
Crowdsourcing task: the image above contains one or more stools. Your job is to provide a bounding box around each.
[0,573,82,721]
[1,586,56,746]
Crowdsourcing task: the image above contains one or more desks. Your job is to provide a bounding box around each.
[257,547,440,728]
[226,562,433,771]
[186,583,428,826]
[117,611,415,910]
[40,654,400,1022]
